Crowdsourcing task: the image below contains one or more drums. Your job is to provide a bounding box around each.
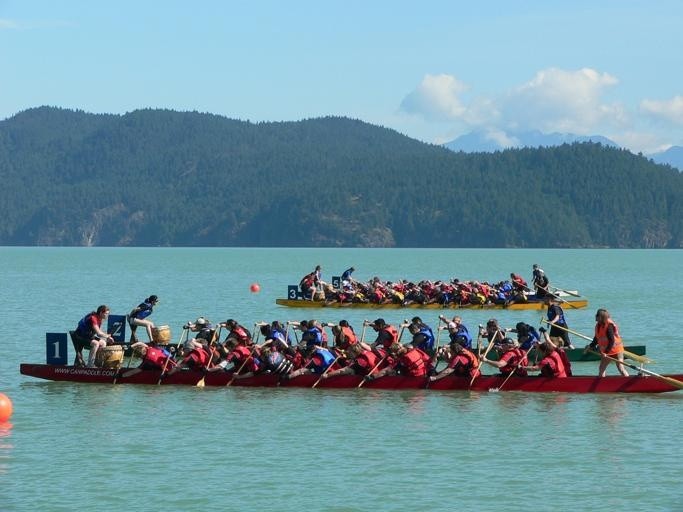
[99,345,124,367]
[152,325,171,345]
[313,291,325,301]
[323,284,333,292]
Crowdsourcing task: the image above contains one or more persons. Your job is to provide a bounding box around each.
[300,264,549,306]
[123,316,573,379]
[128,295,159,342]
[75,305,114,370]
[585,308,629,377]
[543,293,575,349]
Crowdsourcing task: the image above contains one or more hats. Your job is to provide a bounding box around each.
[447,322,457,329]
[194,319,205,325]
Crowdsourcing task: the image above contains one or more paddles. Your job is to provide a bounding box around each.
[196,346,217,387]
[540,316,655,364]
[549,285,581,297]
[535,283,577,309]
[583,345,682,388]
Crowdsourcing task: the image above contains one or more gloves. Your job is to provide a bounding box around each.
[282,374,290,380]
[160,373,168,379]
[200,366,209,374]
[364,375,373,381]
[322,323,328,327]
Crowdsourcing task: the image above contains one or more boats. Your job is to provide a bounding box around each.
[18,363,683,393]
